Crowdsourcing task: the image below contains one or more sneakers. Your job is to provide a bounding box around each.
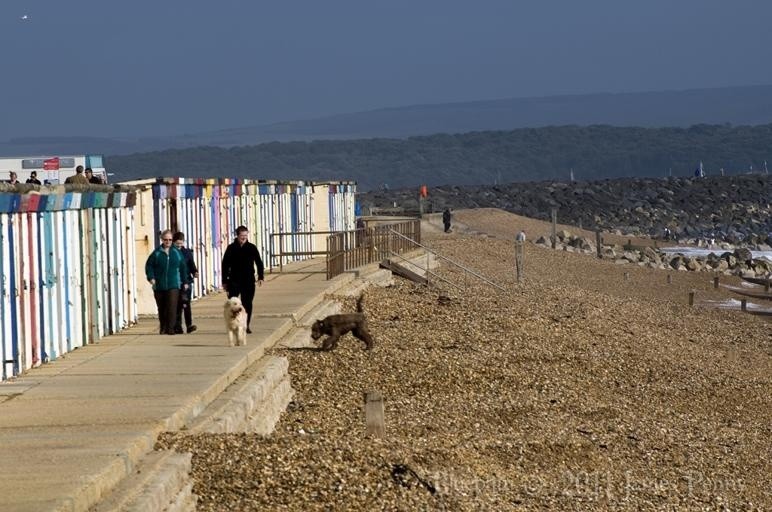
[176,328,183,335]
[159,327,167,335]
[186,326,197,334]
[245,328,252,333]
[167,329,175,335]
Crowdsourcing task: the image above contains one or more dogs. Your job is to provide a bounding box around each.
[222,295,250,348]
[310,293,374,350]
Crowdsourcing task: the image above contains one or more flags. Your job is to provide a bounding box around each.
[43,157,60,170]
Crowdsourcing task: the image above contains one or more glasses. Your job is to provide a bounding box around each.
[163,239,172,242]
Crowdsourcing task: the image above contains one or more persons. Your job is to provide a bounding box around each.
[171,231,201,334]
[357,216,365,228]
[442,207,454,234]
[0,164,109,186]
[145,229,191,336]
[221,225,265,334]
[515,230,527,242]
